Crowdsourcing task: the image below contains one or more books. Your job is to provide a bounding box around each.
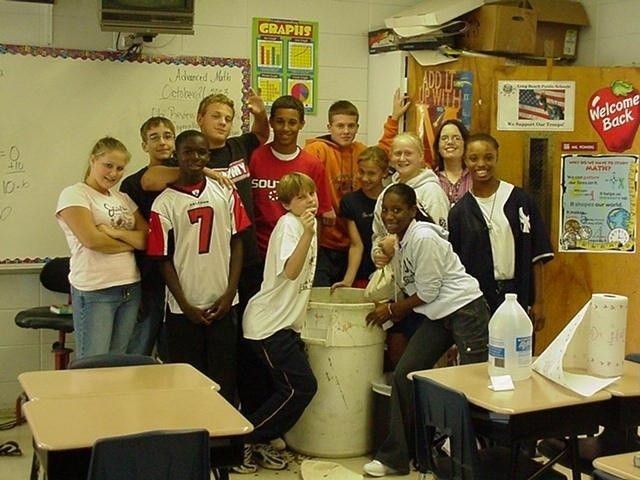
[50,304,74,315]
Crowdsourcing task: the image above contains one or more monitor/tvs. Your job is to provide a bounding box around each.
[100,0,197,36]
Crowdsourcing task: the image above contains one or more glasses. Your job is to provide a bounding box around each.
[148,133,174,142]
[440,136,462,144]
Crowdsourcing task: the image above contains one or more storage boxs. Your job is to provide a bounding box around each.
[461,3,537,53]
[531,0,593,62]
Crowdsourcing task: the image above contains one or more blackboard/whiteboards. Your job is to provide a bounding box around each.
[0,43,251,275]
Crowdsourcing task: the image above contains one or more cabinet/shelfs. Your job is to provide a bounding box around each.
[367,51,425,139]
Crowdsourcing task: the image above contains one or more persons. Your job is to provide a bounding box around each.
[119,85,493,478]
[55,137,149,360]
[447,134,555,449]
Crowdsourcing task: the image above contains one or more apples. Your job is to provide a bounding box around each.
[588,79,640,152]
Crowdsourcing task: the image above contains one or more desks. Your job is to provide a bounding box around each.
[19,390,253,479]
[592,452,640,479]
[407,356,611,480]
[16,363,221,398]
[567,361,640,453]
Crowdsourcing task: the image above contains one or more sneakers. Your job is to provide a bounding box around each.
[252,443,288,470]
[271,438,286,450]
[362,459,400,477]
[232,444,257,474]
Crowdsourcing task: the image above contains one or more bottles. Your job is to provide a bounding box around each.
[487,294,533,382]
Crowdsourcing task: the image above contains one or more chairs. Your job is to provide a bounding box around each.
[412,375,561,480]
[14,255,75,371]
[70,353,155,369]
[88,428,211,479]
[538,353,640,475]
